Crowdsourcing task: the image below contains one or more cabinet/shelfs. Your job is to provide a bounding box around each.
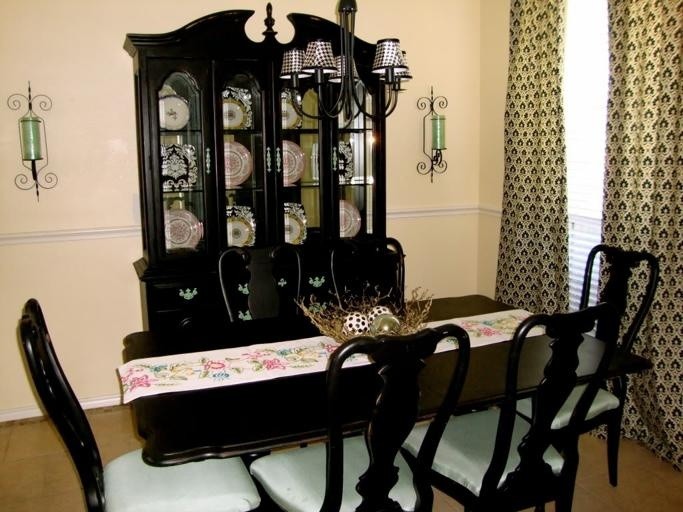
[135,278,405,332]
[120,1,405,284]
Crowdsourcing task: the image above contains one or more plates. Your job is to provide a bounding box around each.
[155,94,362,252]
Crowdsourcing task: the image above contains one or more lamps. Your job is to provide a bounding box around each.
[7,81,58,202]
[416,86,447,184]
[279,1,413,121]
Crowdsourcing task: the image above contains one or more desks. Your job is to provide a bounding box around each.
[121,293,654,467]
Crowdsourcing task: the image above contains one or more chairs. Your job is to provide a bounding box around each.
[328,233,405,313]
[21,299,263,512]
[511,244,660,490]
[396,301,623,512]
[217,238,303,469]
[249,321,471,512]
